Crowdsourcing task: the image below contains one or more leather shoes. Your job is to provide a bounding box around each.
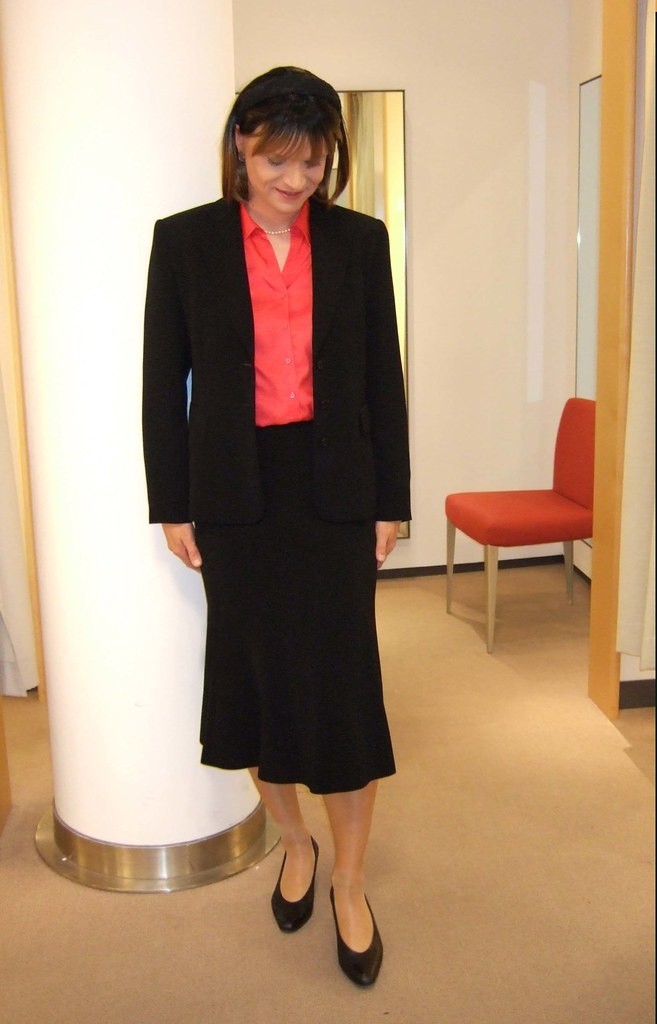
[330,885,384,987]
[270,835,319,933]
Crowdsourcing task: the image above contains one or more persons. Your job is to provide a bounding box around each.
[142,62,412,986]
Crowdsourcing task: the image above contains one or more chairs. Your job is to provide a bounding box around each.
[446,397,596,654]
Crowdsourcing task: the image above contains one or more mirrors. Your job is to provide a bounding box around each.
[326,88,409,410]
[577,74,602,548]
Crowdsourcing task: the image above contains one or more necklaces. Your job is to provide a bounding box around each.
[264,227,291,235]
